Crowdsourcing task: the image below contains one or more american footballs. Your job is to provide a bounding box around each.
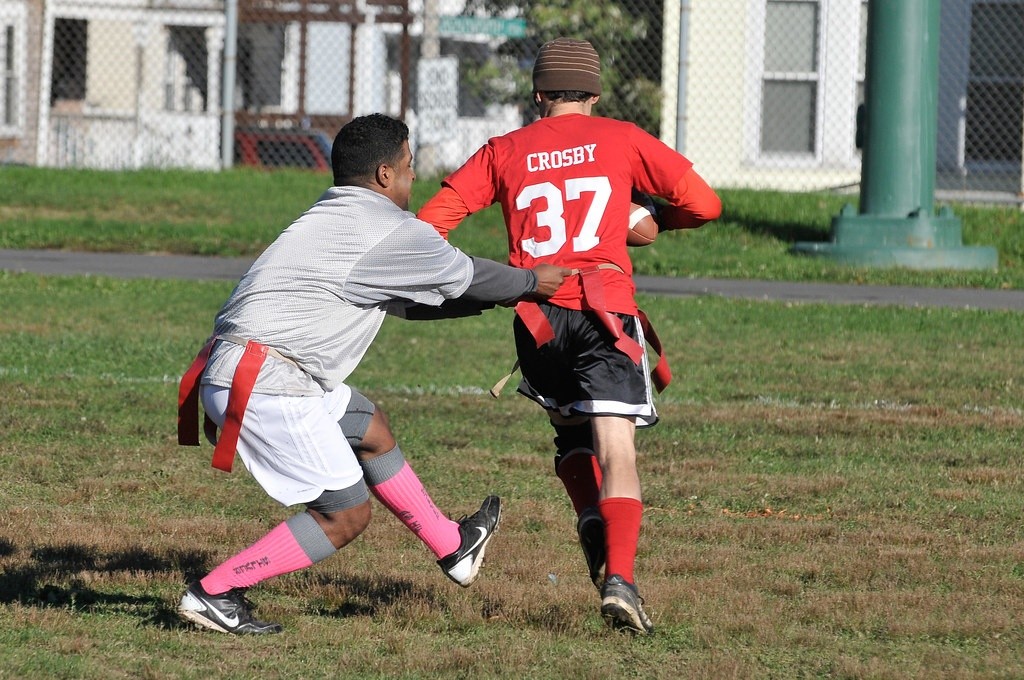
[626,185,660,248]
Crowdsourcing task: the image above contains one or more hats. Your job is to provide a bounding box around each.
[532,37,601,96]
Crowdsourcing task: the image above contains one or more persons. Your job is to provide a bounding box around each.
[416,38,721,637]
[178,112,572,637]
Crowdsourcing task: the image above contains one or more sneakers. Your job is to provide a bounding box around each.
[435,495,502,588]
[175,579,282,638]
[600,574,654,638]
